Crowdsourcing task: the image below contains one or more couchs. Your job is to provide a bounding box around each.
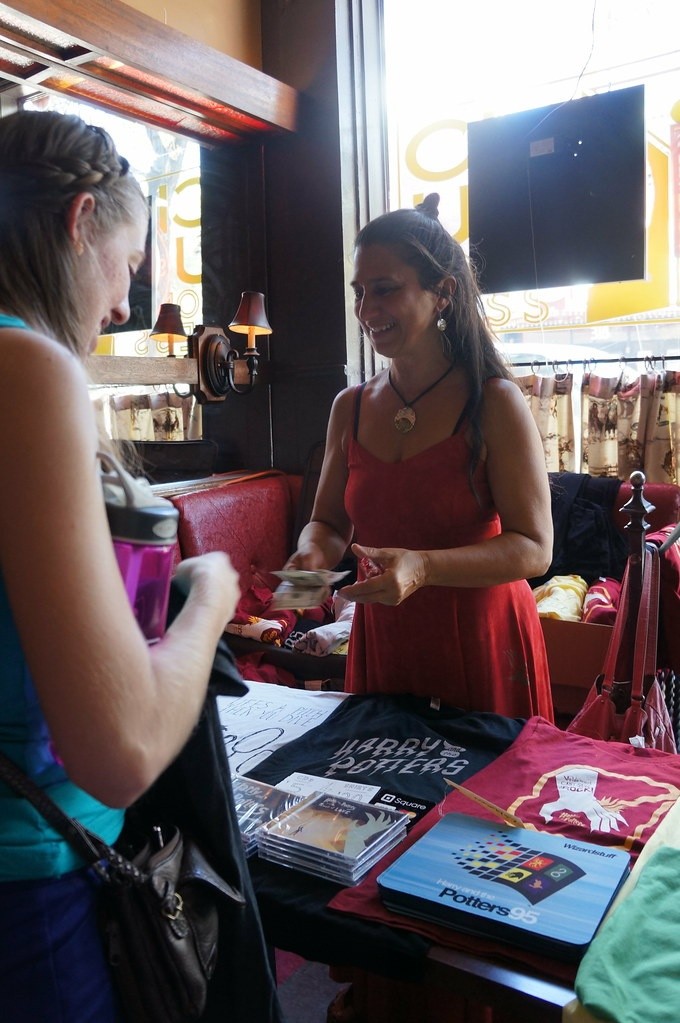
[167,468,680,723]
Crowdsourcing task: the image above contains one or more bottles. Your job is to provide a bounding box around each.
[49,476,177,765]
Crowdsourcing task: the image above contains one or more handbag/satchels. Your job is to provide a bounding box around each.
[97,814,244,1023]
[568,546,677,753]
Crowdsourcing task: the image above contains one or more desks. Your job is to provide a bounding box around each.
[209,679,680,1023]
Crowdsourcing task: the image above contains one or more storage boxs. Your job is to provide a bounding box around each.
[530,565,622,692]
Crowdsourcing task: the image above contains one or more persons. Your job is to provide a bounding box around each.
[282,191,556,731]
[0,109,247,1022]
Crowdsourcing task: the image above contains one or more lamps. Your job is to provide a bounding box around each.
[194,291,274,403]
[149,303,193,398]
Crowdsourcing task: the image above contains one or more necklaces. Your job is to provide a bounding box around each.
[387,364,455,433]
